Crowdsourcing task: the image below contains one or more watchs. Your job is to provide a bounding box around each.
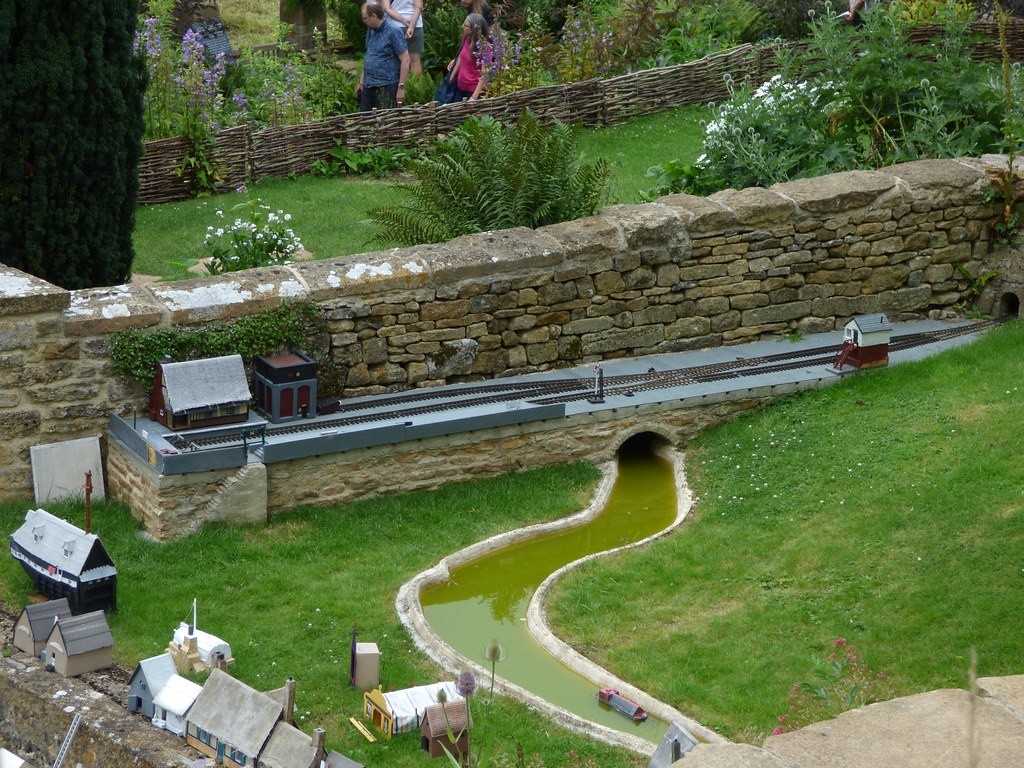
[398,83,405,89]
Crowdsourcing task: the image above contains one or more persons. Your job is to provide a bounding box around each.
[381,0,423,75]
[846,0,875,31]
[357,2,410,116]
[463,0,495,30]
[448,13,492,102]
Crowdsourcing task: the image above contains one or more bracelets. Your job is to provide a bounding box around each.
[472,95,477,100]
[358,82,364,87]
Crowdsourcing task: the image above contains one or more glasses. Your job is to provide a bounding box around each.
[462,21,473,29]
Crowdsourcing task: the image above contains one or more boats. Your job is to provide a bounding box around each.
[598,687,648,722]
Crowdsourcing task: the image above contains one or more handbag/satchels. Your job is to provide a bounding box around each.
[432,36,467,107]
